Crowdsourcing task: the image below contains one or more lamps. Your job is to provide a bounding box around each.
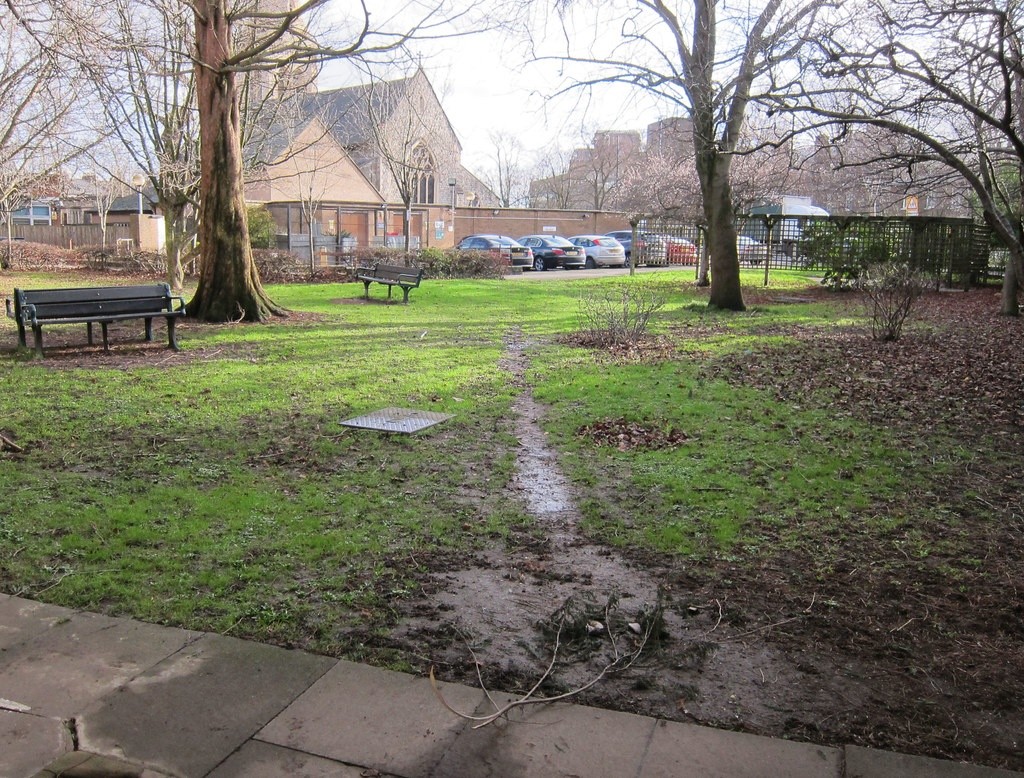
[381,204,386,209]
[583,214,590,218]
[493,210,501,215]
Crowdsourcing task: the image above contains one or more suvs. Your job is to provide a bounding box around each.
[601,230,668,268]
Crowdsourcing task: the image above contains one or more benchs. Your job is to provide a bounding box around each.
[5,283,187,358]
[355,261,422,303]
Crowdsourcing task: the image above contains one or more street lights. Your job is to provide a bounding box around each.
[447,177,456,247]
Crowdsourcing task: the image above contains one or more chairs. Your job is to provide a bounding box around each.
[581,242,591,247]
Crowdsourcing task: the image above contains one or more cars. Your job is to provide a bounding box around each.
[453,233,534,271]
[565,235,626,269]
[658,235,698,266]
[514,235,586,271]
[737,235,767,266]
